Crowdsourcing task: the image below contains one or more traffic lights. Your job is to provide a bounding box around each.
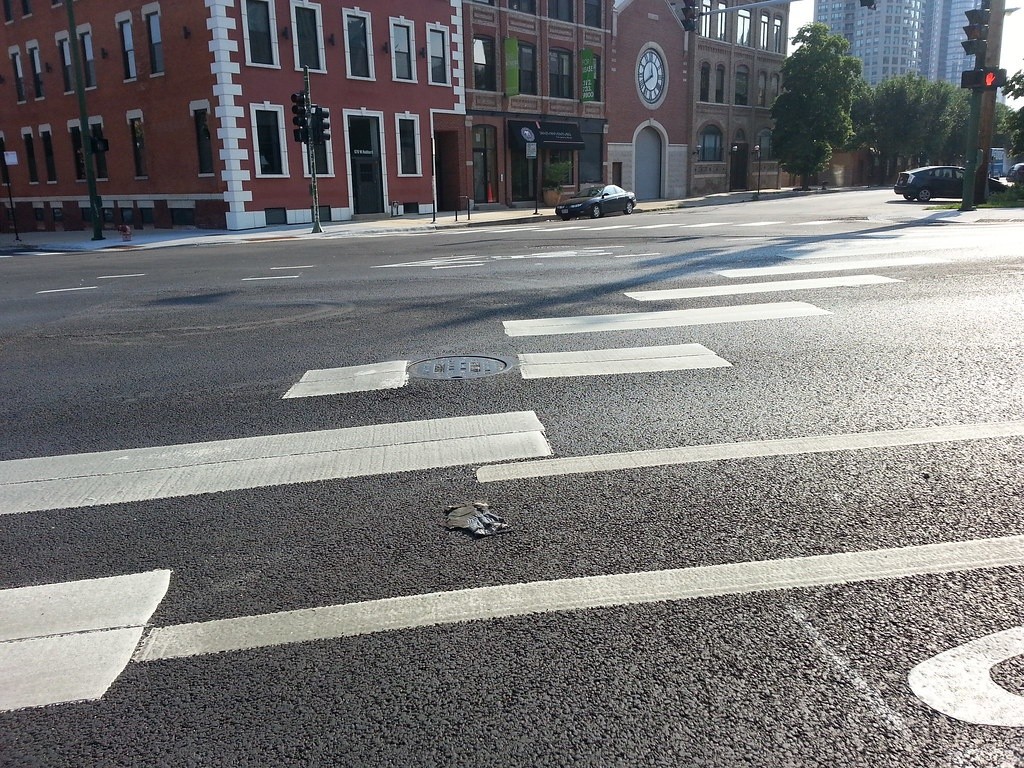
[960,8,1006,92]
[311,104,331,145]
[290,92,309,143]
[681,0,696,32]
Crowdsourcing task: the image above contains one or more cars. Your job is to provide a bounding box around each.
[894,166,1008,202]
[1007,162,1024,183]
[555,184,637,221]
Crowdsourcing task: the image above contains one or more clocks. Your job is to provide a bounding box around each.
[638,47,667,105]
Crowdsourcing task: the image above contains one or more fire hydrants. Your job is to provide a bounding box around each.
[117,225,131,241]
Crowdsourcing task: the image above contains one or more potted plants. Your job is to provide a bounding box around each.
[540,160,572,206]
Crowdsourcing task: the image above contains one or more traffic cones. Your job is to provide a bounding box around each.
[488,182,493,203]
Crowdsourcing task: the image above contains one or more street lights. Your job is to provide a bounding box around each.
[754,145,762,196]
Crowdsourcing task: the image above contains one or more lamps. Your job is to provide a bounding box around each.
[692,144,701,155]
[728,145,739,155]
